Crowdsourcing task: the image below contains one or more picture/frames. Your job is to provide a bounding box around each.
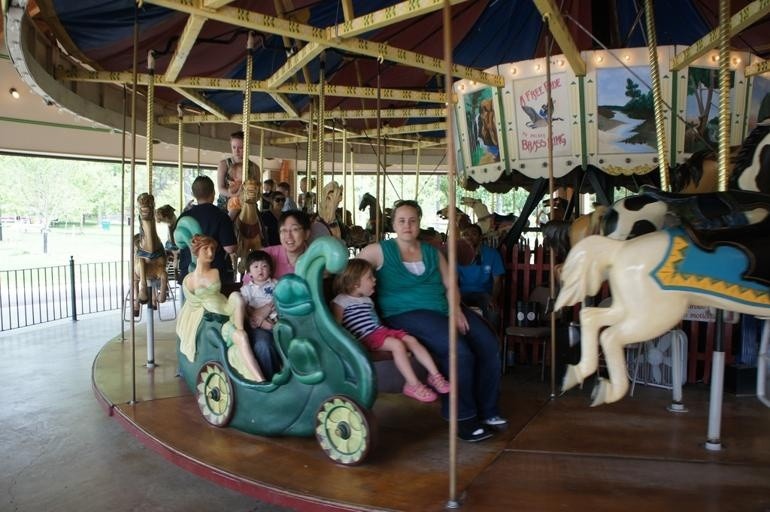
[452,65,503,184]
[497,54,582,179]
[744,54,770,139]
[582,43,674,177]
[674,45,749,195]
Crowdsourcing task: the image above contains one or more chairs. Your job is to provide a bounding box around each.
[500,286,552,386]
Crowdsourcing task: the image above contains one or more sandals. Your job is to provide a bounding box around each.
[482,415,509,429]
[443,418,494,443]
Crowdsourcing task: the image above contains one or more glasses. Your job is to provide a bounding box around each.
[279,226,304,234]
[275,198,286,202]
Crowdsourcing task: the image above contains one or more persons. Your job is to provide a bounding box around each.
[330,259,451,404]
[217,131,260,215]
[239,250,278,334]
[226,163,242,221]
[444,224,506,330]
[172,177,238,294]
[356,199,509,443]
[259,176,318,246]
[242,210,312,381]
[176,233,267,382]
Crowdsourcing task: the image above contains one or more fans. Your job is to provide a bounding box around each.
[626,330,687,390]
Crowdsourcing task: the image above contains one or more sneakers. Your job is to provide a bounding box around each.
[402,383,437,402]
[427,372,449,394]
